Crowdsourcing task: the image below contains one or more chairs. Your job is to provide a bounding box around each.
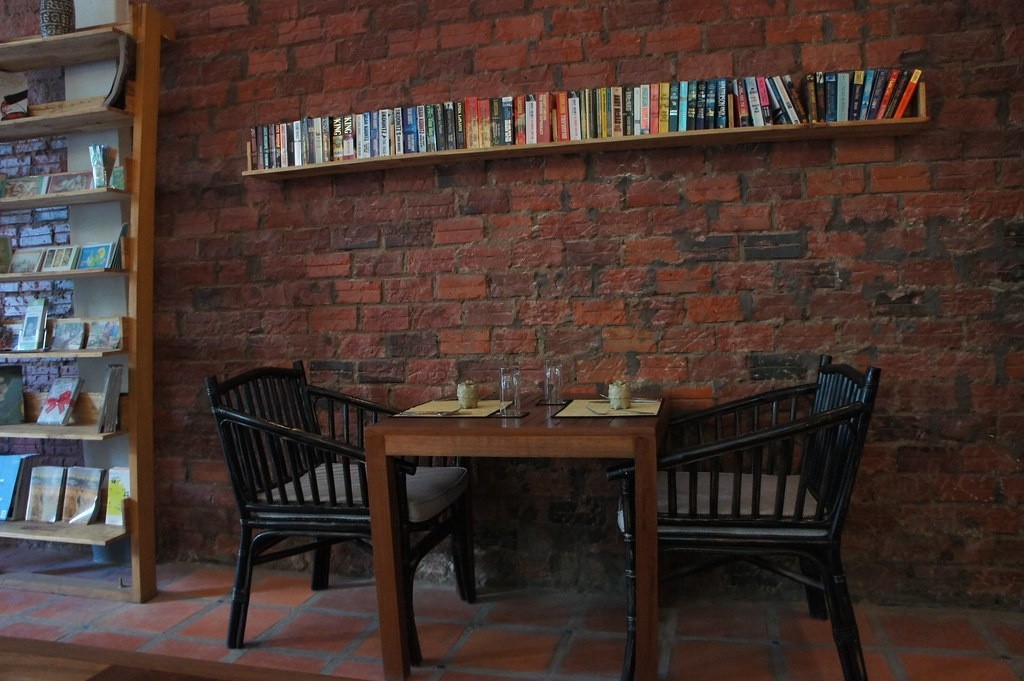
[205,360,475,667]
[605,355,881,680]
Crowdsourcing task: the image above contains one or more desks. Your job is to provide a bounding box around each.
[364,394,666,681]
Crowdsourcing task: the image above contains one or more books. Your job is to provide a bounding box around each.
[251,68,923,170]
[0,35,136,523]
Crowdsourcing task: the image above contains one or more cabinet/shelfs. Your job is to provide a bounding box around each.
[0,2,158,605]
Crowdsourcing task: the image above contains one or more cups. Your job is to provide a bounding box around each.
[498,366,521,417]
[542,359,564,404]
[608,383,632,409]
[456,383,478,409]
